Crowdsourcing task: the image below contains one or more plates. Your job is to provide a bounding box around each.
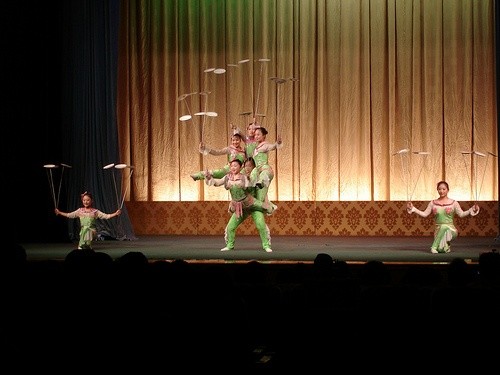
[126,165,139,169]
[177,90,212,102]
[473,151,487,157]
[194,112,206,116]
[61,164,71,167]
[206,112,218,116]
[204,68,216,73]
[414,152,431,155]
[239,59,250,64]
[227,65,240,67]
[487,151,498,157]
[213,69,226,74]
[115,164,128,169]
[460,152,472,154]
[179,115,192,121]
[239,77,300,117]
[103,163,115,169]
[392,149,411,157]
[44,165,57,168]
[258,59,271,62]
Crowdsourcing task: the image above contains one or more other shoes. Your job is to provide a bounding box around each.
[265,247,272,252]
[221,247,231,251]
[430,249,438,253]
[267,206,277,216]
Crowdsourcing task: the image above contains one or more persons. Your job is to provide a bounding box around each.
[190,121,284,252]
[55,191,122,249]
[0,237,500,374]
[406,181,479,253]
